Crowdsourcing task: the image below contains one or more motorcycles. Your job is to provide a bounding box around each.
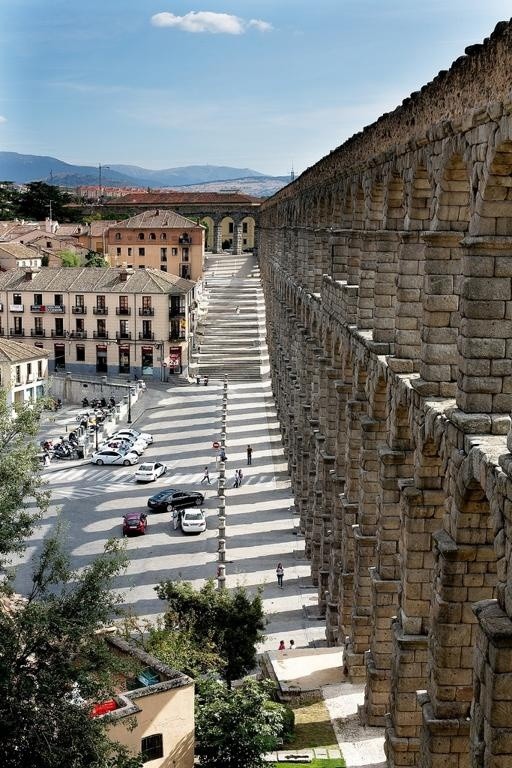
[80,395,115,408]
[41,435,77,461]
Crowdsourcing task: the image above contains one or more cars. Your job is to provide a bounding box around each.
[89,427,207,539]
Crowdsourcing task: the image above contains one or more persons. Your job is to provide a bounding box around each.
[276,562,284,588]
[290,640,296,649]
[247,445,252,464]
[238,469,243,486]
[233,469,239,488]
[221,448,225,461]
[278,640,285,650]
[201,466,212,484]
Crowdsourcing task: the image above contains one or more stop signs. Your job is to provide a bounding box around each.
[213,442,219,449]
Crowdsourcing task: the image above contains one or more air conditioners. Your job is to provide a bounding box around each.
[97,309,104,314]
[144,309,150,315]
[77,332,83,338]
[74,307,82,313]
